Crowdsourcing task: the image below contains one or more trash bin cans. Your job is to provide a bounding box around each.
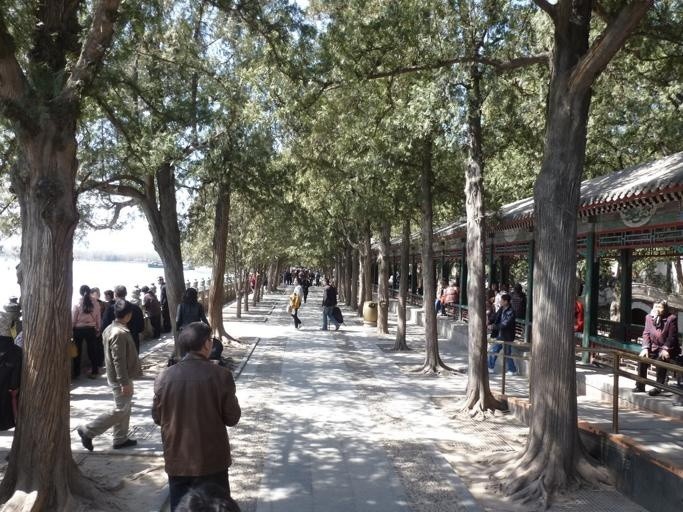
[362,301,378,327]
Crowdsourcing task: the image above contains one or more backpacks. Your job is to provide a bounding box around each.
[332,307,345,326]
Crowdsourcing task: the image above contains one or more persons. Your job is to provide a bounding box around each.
[8,330,24,426]
[435,278,527,316]
[488,294,518,375]
[72,276,172,452]
[574,300,584,333]
[631,298,679,396]
[175,287,210,331]
[151,322,241,512]
[283,268,341,331]
[173,482,241,512]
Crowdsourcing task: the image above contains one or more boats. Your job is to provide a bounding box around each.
[182,261,195,270]
[147,260,163,269]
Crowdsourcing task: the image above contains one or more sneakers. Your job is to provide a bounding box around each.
[71,366,106,380]
[77,429,93,453]
[320,324,340,331]
[296,322,304,330]
[113,439,137,447]
[505,371,518,376]
[631,387,645,394]
[650,388,662,397]
[485,367,494,373]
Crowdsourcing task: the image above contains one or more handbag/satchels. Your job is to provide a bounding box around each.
[286,304,297,317]
[69,342,79,358]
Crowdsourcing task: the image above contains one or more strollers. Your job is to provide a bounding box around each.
[208,335,225,368]
